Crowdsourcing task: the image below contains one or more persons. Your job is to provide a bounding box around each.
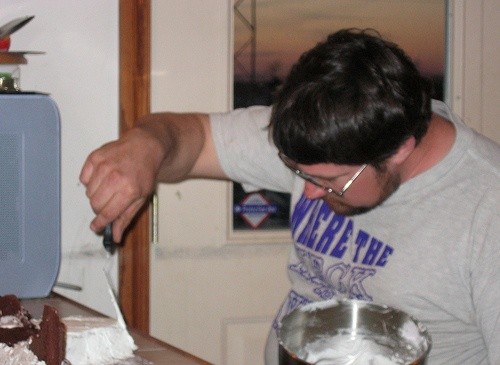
[80,28,500,365]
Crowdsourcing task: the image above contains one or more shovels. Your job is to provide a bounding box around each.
[99,222,140,350]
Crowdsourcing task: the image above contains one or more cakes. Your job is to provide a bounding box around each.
[31,315,138,365]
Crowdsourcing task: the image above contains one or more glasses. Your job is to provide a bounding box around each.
[278,151,367,197]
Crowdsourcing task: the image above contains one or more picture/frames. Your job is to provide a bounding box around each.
[229,0,452,239]
